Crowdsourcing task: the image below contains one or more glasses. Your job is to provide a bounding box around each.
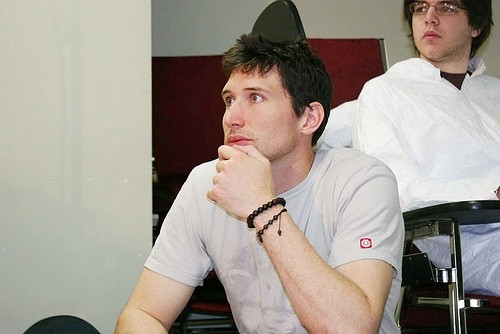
[405,3,470,17]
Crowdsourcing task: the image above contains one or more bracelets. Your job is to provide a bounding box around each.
[247,197,286,227]
[257,209,288,243]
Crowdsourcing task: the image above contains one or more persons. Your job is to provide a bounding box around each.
[112,31,406,334]
[313,0,500,297]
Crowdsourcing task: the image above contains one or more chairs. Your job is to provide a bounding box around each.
[249,1,500,334]
